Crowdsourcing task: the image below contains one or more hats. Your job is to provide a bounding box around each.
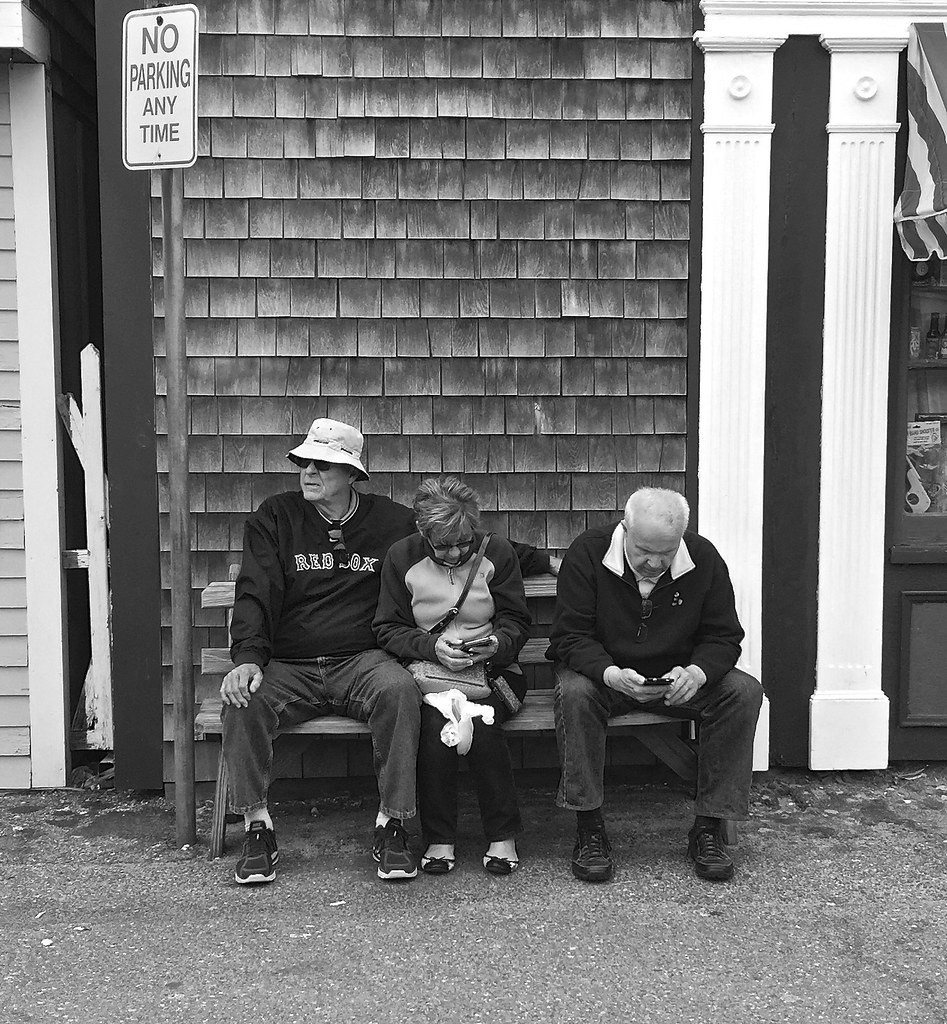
[285,418,370,481]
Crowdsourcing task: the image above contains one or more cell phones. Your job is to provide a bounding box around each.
[459,638,492,652]
[641,677,675,687]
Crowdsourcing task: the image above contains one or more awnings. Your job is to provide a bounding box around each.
[894,21,946,261]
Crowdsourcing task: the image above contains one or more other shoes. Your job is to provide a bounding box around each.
[421,844,457,872]
[482,839,520,874]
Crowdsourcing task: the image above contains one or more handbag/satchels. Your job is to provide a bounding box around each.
[405,659,492,700]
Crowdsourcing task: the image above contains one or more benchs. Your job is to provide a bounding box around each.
[193,564,738,862]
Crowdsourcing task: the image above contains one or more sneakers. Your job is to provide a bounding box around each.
[572,821,612,880]
[684,826,733,878]
[235,820,279,883]
[372,817,418,878]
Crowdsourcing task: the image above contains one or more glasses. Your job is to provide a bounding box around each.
[297,457,331,471]
[328,520,349,565]
[633,598,653,643]
[427,533,474,551]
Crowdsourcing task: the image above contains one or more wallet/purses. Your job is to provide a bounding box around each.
[492,676,523,713]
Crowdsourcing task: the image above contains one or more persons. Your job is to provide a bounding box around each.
[543,487,764,882]
[372,473,533,876]
[219,417,564,884]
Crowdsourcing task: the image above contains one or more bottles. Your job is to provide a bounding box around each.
[939,317,947,359]
[927,312,941,360]
